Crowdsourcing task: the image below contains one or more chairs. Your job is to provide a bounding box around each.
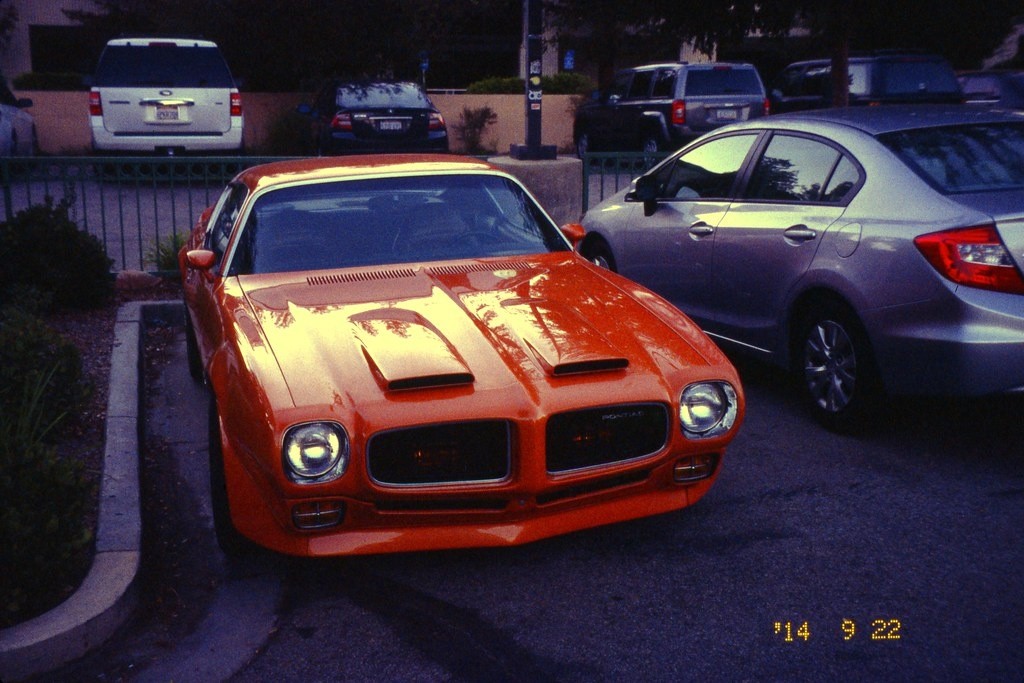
[391,204,479,256]
[255,211,335,275]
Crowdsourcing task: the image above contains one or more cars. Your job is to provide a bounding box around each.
[296,81,450,154]
[578,106,1024,434]
[176,152,746,557]
[0,78,41,156]
[957,70,1024,105]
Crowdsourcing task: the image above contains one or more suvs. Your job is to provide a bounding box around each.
[768,54,968,105]
[86,37,244,154]
[572,60,769,174]
